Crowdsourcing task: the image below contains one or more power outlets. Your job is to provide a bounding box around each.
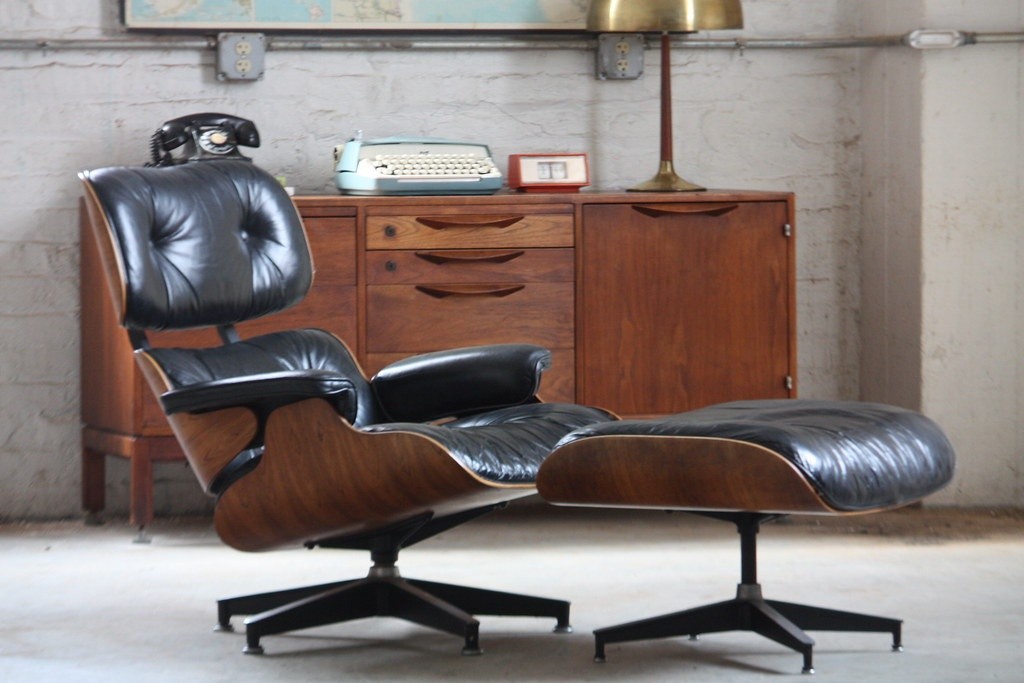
[596,32,646,81]
[214,30,264,81]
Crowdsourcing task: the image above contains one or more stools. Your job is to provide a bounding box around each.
[537,399,958,676]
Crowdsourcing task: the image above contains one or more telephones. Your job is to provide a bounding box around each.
[149,111,261,165]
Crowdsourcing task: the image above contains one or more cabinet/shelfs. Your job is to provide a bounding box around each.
[79,191,797,545]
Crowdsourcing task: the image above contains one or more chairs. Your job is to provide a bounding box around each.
[78,159,621,657]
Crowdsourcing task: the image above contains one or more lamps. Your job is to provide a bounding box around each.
[584,0,745,191]
